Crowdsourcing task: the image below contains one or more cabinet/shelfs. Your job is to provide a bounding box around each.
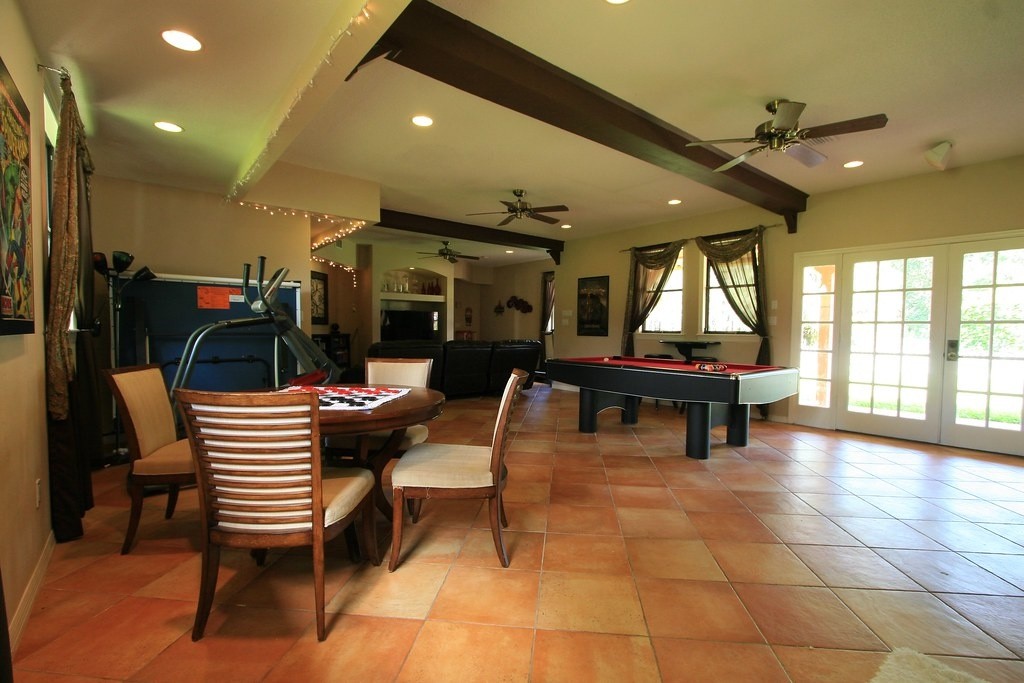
[311,333,351,368]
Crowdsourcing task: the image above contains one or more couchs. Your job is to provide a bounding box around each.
[367,338,543,401]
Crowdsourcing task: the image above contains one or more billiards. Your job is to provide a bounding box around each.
[695,364,728,372]
[603,358,609,362]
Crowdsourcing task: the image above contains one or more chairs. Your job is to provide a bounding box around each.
[324,357,434,515]
[387,368,529,573]
[97,362,197,556]
[173,384,380,644]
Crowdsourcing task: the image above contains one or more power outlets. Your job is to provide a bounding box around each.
[36,478,42,509]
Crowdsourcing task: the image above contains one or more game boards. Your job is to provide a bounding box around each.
[268,385,412,410]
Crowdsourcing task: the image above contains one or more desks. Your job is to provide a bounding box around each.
[659,339,722,360]
[242,383,446,566]
[545,356,800,460]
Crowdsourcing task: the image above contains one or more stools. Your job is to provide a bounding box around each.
[679,356,719,415]
[645,352,677,410]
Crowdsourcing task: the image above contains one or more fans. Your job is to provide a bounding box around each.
[415,241,480,263]
[465,189,570,226]
[686,98,889,173]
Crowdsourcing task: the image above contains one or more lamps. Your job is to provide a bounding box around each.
[923,140,957,171]
[92,250,158,466]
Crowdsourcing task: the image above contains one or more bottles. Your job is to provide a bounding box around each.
[410,273,419,293]
[380,279,402,292]
[402,274,408,293]
[419,278,441,295]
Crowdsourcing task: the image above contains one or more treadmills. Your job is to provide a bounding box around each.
[161,254,347,440]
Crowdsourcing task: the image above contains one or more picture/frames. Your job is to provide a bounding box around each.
[576,275,610,338]
[311,270,330,325]
